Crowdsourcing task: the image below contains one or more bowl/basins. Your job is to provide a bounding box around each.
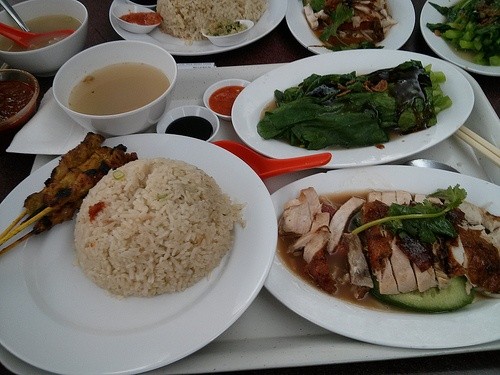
[53,39,176,136]
[0,67,40,131]
[0,0,89,74]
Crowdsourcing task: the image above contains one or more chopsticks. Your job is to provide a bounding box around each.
[453,125,500,166]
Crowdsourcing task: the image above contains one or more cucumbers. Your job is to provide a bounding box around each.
[372,276,477,313]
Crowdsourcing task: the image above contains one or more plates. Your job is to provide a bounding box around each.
[202,18,254,47]
[109,0,288,56]
[286,0,417,54]
[156,105,219,144]
[0,132,279,375]
[231,50,474,169]
[262,164,499,349]
[111,3,163,34]
[420,0,500,77]
[125,0,157,11]
[203,78,252,120]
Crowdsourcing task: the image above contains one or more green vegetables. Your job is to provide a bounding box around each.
[426,0,500,67]
[302,0,384,51]
[353,184,468,244]
[257,59,452,151]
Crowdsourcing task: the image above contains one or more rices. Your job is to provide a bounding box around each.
[156,0,270,40]
[75,158,246,297]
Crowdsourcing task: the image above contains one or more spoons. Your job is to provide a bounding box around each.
[0,22,77,49]
[213,139,333,179]
[403,159,462,173]
